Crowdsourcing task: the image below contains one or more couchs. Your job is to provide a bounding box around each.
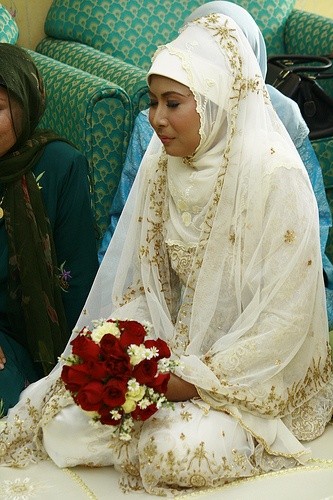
[0,0,333,241]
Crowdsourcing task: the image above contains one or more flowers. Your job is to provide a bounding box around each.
[57,316,173,443]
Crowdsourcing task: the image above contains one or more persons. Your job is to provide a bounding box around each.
[98,0,333,329]
[0,15,333,497]
[0,42,98,417]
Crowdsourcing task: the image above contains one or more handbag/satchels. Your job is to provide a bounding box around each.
[266,55,333,132]
